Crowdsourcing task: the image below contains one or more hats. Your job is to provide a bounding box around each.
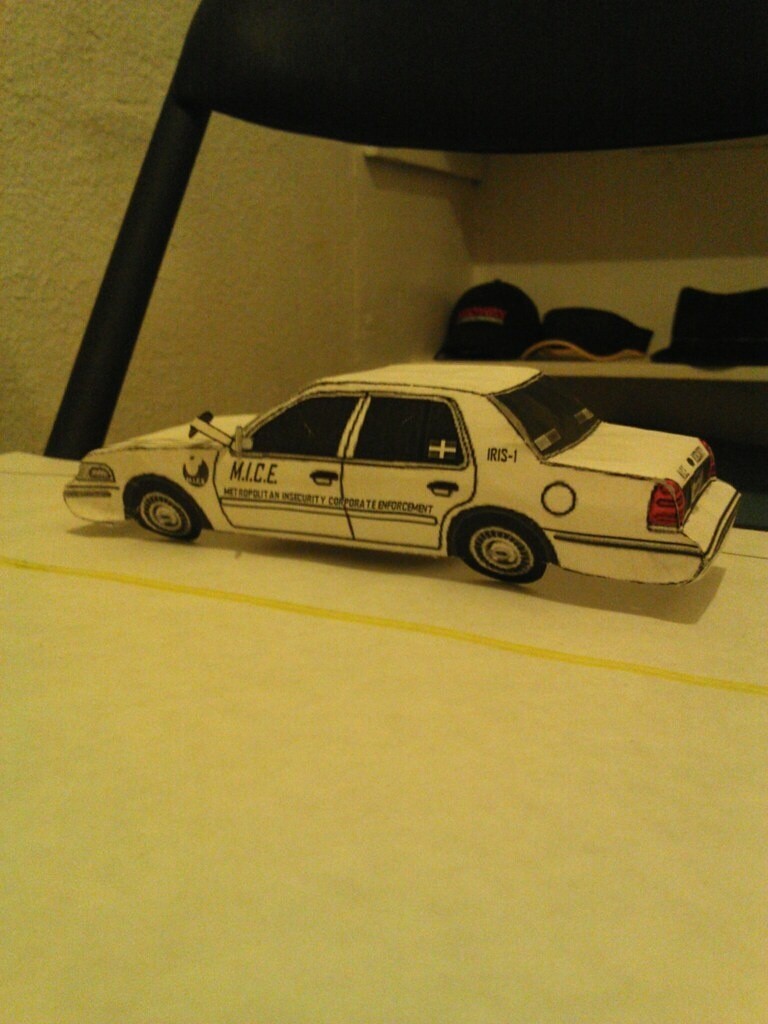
[433,278,538,363]
[655,285,768,370]
[522,302,653,363]
